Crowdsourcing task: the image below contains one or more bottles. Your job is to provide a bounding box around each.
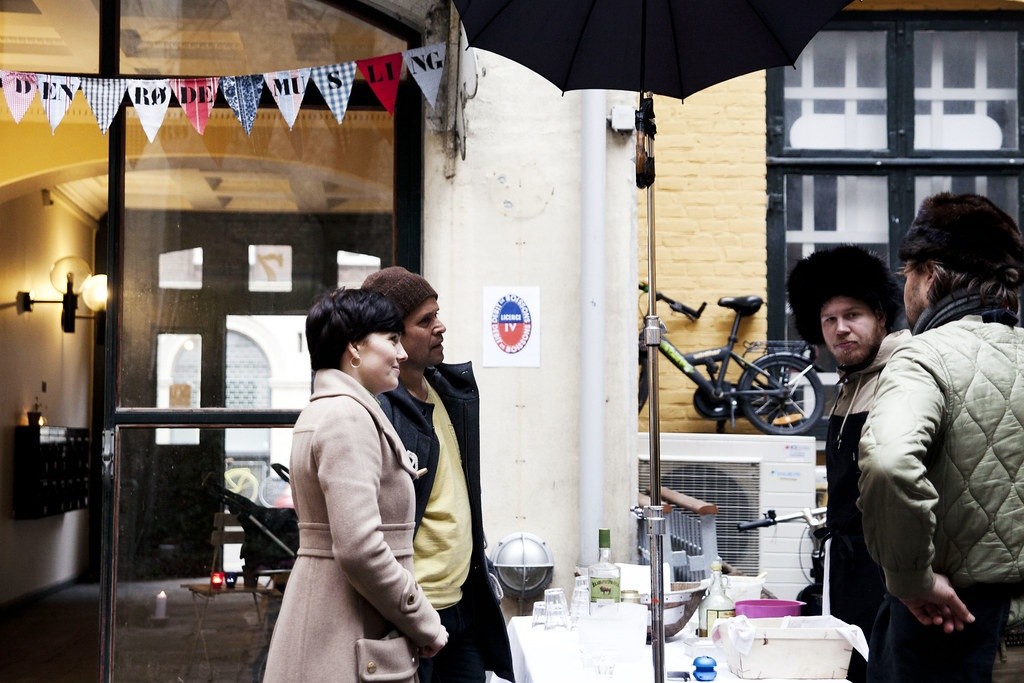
[698,561,736,637]
[587,527,621,615]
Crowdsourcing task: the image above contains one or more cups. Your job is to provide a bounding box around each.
[224,572,239,588]
[211,572,225,589]
[533,574,648,682]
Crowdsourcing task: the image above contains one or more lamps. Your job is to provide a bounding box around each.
[23,254,108,332]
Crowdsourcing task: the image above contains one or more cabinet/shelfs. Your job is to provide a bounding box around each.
[13,425,90,520]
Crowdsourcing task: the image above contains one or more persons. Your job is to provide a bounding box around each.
[857,191,1024,683]
[262,287,451,683]
[362,266,515,683]
[787,242,912,683]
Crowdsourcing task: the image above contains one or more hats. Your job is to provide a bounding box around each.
[901,194,1024,289]
[788,246,904,346]
[361,265,438,319]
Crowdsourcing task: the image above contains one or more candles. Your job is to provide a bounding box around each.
[156,591,167,618]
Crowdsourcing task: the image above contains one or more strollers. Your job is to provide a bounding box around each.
[203,471,300,593]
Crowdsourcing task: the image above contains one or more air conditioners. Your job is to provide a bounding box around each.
[639,434,817,601]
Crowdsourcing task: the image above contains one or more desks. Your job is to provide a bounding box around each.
[490,616,855,683]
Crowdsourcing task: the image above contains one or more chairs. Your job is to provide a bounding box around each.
[180,513,271,683]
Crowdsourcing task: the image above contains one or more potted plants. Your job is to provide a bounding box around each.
[26,396,49,425]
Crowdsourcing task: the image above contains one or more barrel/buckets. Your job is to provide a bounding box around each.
[701,575,766,602]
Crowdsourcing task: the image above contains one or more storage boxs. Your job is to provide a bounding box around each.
[718,616,853,679]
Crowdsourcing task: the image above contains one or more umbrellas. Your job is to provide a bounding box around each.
[452,0,863,683]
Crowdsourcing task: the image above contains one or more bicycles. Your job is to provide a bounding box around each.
[637,283,827,438]
[736,505,827,617]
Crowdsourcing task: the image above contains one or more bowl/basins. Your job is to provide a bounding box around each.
[622,581,707,637]
[735,599,807,618]
